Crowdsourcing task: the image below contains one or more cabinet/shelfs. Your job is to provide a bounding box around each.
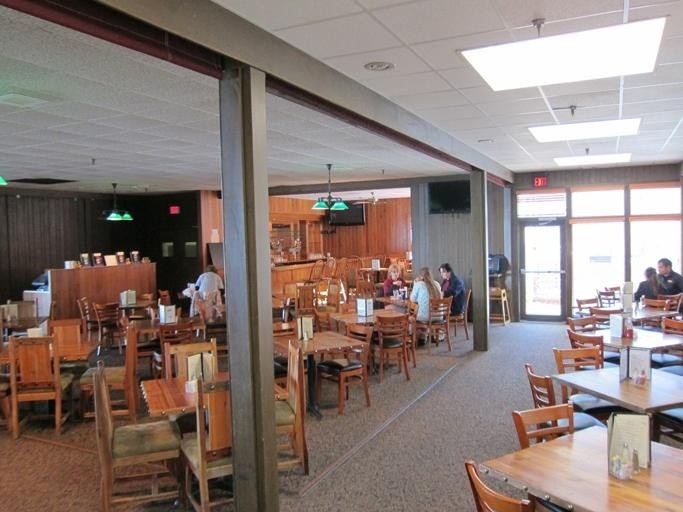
[22,261,157,324]
[272,256,373,295]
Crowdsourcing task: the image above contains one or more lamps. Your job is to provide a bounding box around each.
[310,165,350,210]
[170,206,180,214]
[97,183,134,220]
[0,176,9,186]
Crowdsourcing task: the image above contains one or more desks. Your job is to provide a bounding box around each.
[480,425,683,512]
[374,292,446,313]
[134,318,207,345]
[0,338,100,413]
[574,328,683,360]
[329,310,406,333]
[359,267,388,282]
[552,367,683,414]
[141,372,289,416]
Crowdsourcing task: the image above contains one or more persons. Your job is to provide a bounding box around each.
[196,264,224,301]
[410,267,445,346]
[657,258,683,295]
[634,267,670,301]
[439,263,466,316]
[150,289,181,317]
[384,264,406,297]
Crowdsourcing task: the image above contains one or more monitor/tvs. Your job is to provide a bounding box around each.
[328,200,366,227]
[427,180,471,214]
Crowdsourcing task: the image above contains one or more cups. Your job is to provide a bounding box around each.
[393,289,399,299]
[400,289,406,303]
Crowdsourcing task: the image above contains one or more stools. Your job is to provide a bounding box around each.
[489,289,510,326]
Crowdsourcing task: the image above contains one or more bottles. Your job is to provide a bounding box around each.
[304,329,309,342]
[632,368,639,385]
[639,369,646,384]
[618,442,632,480]
[625,319,633,338]
[632,448,641,474]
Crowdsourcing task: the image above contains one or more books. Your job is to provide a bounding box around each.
[622,294,633,308]
[26,319,53,351]
[605,411,654,473]
[297,316,314,341]
[120,290,136,306]
[372,259,379,269]
[358,298,373,316]
[623,282,632,292]
[619,346,652,382]
[159,304,176,324]
[610,312,630,337]
[2,304,19,322]
[187,350,216,385]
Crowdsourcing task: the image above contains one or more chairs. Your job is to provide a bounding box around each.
[552,345,624,420]
[653,407,683,447]
[92,360,184,511]
[79,320,138,422]
[446,286,473,340]
[179,375,234,512]
[374,252,386,282]
[140,370,290,507]
[151,323,194,377]
[304,260,325,284]
[568,330,620,372]
[347,254,364,286]
[596,290,616,307]
[274,340,310,475]
[8,333,76,438]
[334,258,348,279]
[77,297,114,346]
[637,296,669,327]
[322,257,337,280]
[417,296,453,355]
[312,308,348,360]
[165,339,218,378]
[657,293,683,327]
[8,300,49,334]
[295,283,320,329]
[356,280,374,301]
[577,297,608,330]
[567,315,598,332]
[513,400,573,449]
[320,279,341,332]
[317,325,373,414]
[377,311,410,383]
[464,459,556,512]
[91,301,129,354]
[525,364,607,443]
[651,317,683,365]
[1,304,36,342]
[604,286,622,305]
